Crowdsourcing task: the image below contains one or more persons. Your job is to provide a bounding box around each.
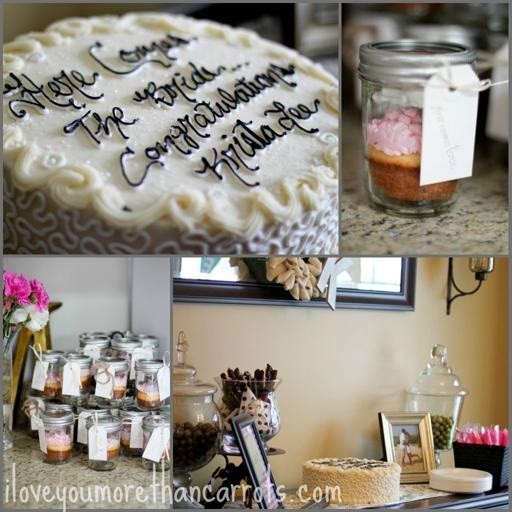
[399,427,409,466]
[404,432,421,465]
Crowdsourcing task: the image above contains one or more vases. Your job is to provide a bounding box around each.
[1,337,15,453]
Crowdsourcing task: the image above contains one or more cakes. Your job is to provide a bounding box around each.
[301,457,401,504]
[3,10,338,255]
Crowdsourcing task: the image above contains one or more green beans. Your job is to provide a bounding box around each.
[432,416,454,450]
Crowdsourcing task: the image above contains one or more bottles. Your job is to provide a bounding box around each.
[213,375,286,457]
[27,328,171,474]
[356,40,482,217]
[172,326,225,509]
[402,343,472,469]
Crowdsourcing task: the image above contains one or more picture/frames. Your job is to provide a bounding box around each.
[377,410,437,484]
[228,413,284,509]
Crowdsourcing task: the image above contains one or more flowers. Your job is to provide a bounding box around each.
[0,271,51,338]
